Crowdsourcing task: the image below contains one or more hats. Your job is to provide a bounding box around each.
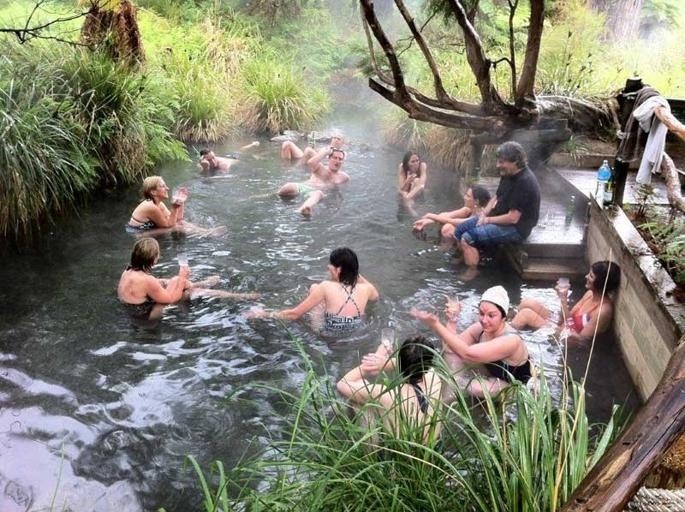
[479,285,510,316]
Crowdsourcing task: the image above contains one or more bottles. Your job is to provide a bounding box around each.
[566,196,577,223]
[596,160,615,192]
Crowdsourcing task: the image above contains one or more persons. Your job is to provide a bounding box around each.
[124,175,227,241]
[117,237,265,320]
[335,338,443,476]
[452,140,541,282]
[411,284,538,412]
[397,150,428,219]
[508,260,620,349]
[278,146,352,215]
[280,132,347,167]
[241,247,378,338]
[413,185,490,239]
[198,140,260,173]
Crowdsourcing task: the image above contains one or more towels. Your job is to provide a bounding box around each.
[616,86,661,163]
[632,94,673,185]
